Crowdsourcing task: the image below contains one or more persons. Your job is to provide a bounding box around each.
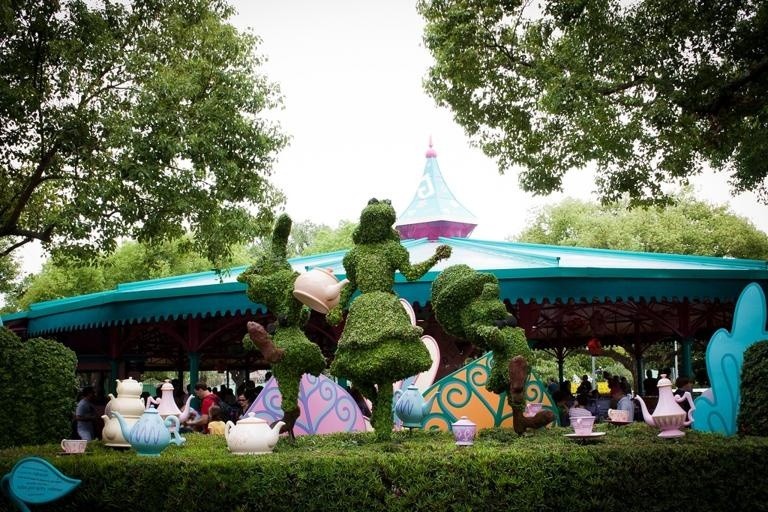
[547,370,694,423]
[325,196,453,440]
[77,389,99,439]
[141,373,264,434]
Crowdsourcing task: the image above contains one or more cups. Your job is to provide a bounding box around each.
[608,408,629,422]
[60,439,88,453]
[451,415,476,446]
[570,415,596,434]
[526,403,544,418]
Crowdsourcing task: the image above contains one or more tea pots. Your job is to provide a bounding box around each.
[101,376,196,458]
[293,266,351,314]
[394,382,441,428]
[634,374,696,439]
[225,411,286,456]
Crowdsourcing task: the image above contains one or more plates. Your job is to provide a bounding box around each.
[563,432,606,438]
